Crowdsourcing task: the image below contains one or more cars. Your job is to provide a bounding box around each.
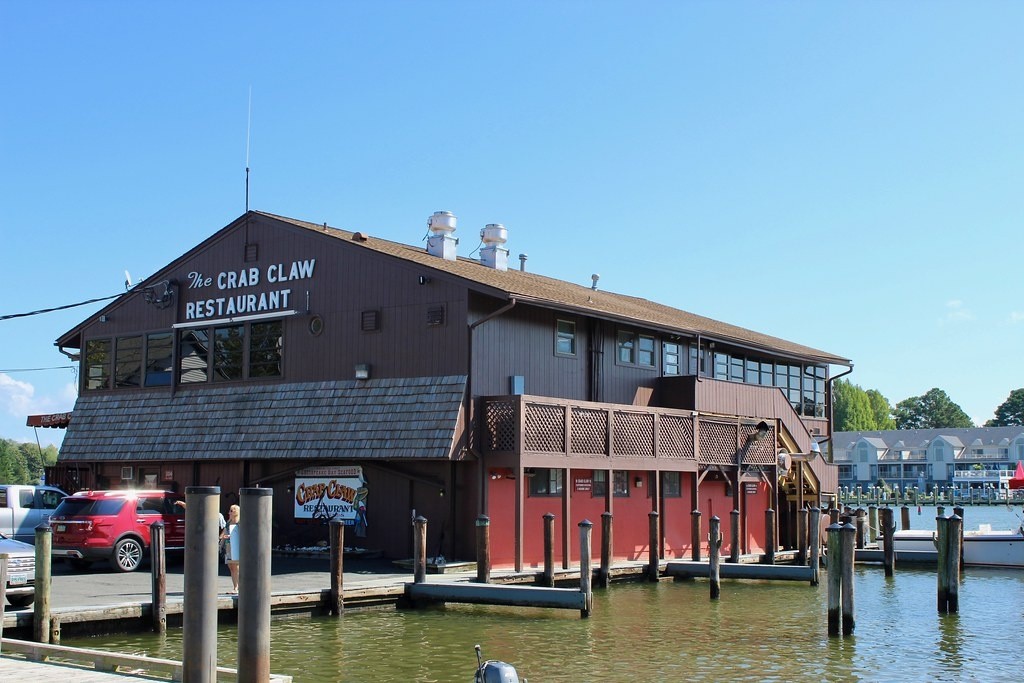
[0,533,39,609]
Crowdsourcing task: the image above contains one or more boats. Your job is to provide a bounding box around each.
[876,510,1024,569]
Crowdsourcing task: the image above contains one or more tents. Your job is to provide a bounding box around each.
[1008,460,1024,490]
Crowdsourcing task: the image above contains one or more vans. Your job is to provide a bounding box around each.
[0,484,71,536]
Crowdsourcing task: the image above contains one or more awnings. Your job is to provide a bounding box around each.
[27,411,74,429]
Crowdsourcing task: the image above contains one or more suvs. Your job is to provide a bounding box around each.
[47,488,188,574]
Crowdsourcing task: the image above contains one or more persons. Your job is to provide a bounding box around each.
[173,501,240,594]
